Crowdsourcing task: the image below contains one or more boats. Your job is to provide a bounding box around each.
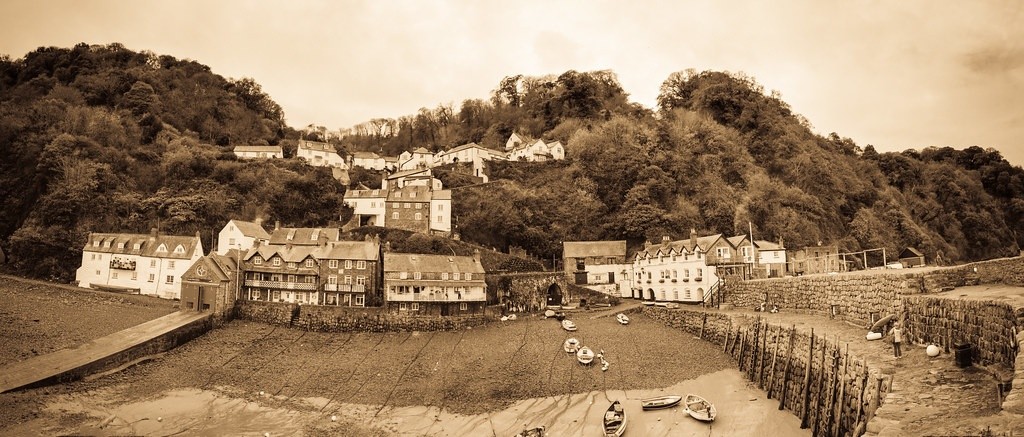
[616,314,629,325]
[546,309,566,320]
[641,395,682,411]
[601,399,627,437]
[562,319,578,331]
[642,301,680,309]
[685,393,717,422]
[514,426,544,437]
[564,338,580,353]
[576,345,594,365]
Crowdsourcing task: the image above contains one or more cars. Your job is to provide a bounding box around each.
[886,262,903,269]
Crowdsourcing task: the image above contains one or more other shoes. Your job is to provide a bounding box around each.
[895,356,901,360]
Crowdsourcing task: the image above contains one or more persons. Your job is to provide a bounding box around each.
[888,322,903,358]
